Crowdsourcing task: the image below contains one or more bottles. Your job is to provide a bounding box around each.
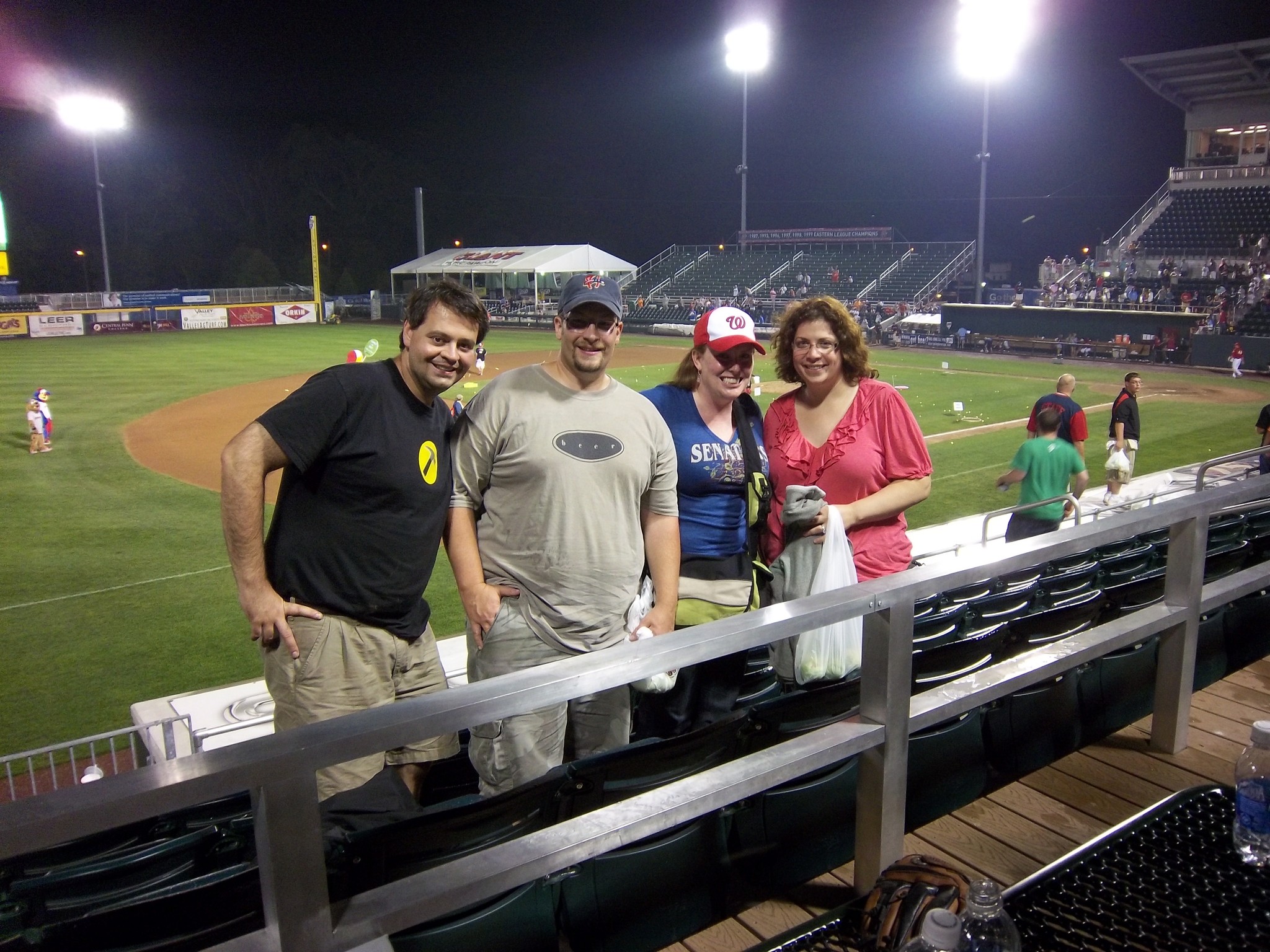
[897,876,1020,952]
[1229,719,1270,868]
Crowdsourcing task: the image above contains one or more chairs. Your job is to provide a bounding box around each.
[2,491,1270,951]
[1078,184,1270,339]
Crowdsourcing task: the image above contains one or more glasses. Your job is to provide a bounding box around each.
[791,340,840,354]
[561,314,620,336]
[1128,381,1143,385]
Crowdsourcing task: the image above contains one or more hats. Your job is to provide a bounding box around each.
[693,306,767,355]
[558,273,623,321]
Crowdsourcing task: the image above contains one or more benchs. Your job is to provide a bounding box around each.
[620,249,967,323]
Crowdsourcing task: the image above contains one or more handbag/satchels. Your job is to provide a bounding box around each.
[794,505,864,686]
[646,552,774,632]
[1104,440,1130,472]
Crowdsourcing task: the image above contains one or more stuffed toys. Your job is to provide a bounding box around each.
[25,399,52,453]
[32,388,52,446]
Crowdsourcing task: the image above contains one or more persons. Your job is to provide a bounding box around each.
[442,271,681,802]
[758,295,934,685]
[1103,372,1143,511]
[623,306,771,740]
[662,294,670,310]
[637,296,643,308]
[1228,342,1245,378]
[1192,132,1267,169]
[993,409,1088,545]
[1255,401,1270,475]
[220,281,489,825]
[495,293,524,316]
[475,342,487,376]
[449,394,465,423]
[108,293,122,307]
[678,220,1269,367]
[1025,373,1088,516]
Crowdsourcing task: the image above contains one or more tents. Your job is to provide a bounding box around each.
[389,242,639,313]
